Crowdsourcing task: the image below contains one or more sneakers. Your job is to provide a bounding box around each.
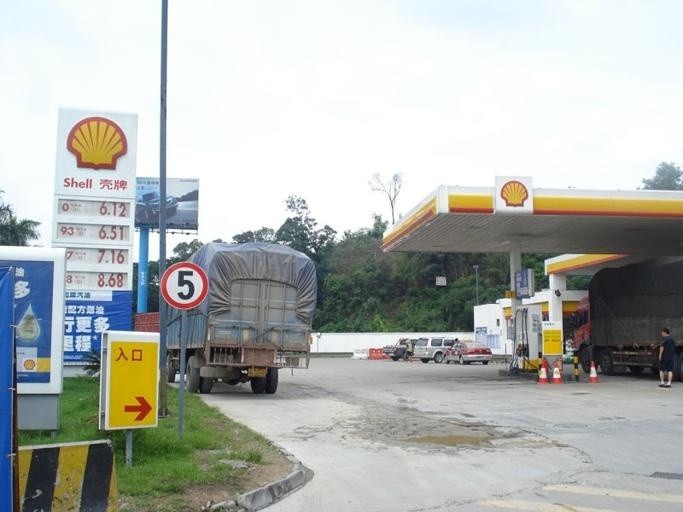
[665,385,671,387]
[658,384,664,387]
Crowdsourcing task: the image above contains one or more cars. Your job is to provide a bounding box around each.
[136,190,180,220]
[444,339,492,365]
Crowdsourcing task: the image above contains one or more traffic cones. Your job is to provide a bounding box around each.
[551,360,564,384]
[587,361,600,384]
[537,359,550,384]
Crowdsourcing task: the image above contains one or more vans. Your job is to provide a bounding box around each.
[412,336,467,363]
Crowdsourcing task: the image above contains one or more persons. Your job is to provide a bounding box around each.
[658,327,675,388]
[451,337,464,350]
[404,337,415,364]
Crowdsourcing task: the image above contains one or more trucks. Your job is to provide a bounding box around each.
[165,243,318,394]
[570,256,682,382]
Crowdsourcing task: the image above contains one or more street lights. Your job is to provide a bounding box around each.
[472,265,480,305]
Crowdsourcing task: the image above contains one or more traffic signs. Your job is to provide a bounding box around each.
[159,261,209,310]
[98,330,160,430]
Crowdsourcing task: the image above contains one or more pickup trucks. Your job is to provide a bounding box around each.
[382,338,417,361]
